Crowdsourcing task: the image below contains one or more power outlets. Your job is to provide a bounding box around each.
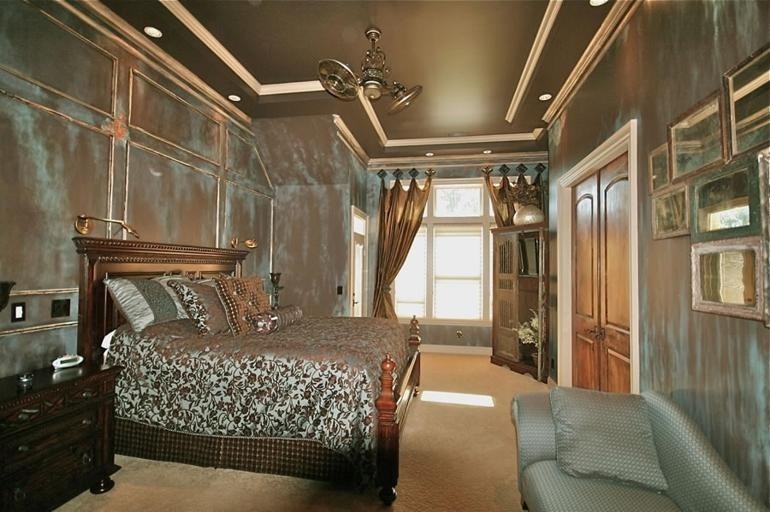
[51,299,70,318]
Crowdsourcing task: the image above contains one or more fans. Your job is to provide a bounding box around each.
[317,58,360,102]
[386,80,423,116]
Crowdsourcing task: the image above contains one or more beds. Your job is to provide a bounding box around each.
[71,237,422,507]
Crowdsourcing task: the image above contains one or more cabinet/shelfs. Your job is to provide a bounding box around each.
[489,222,549,384]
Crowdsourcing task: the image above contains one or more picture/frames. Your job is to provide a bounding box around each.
[647,43,770,328]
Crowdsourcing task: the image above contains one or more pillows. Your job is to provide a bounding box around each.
[102,272,304,340]
[549,386,670,495]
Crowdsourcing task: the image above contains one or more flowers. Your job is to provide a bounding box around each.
[512,309,544,354]
[503,180,547,207]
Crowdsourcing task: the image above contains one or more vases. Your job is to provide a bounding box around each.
[531,352,551,368]
[513,205,544,225]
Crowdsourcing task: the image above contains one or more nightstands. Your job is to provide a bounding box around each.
[0,364,126,512]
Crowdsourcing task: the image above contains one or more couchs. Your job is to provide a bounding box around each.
[511,390,770,512]
